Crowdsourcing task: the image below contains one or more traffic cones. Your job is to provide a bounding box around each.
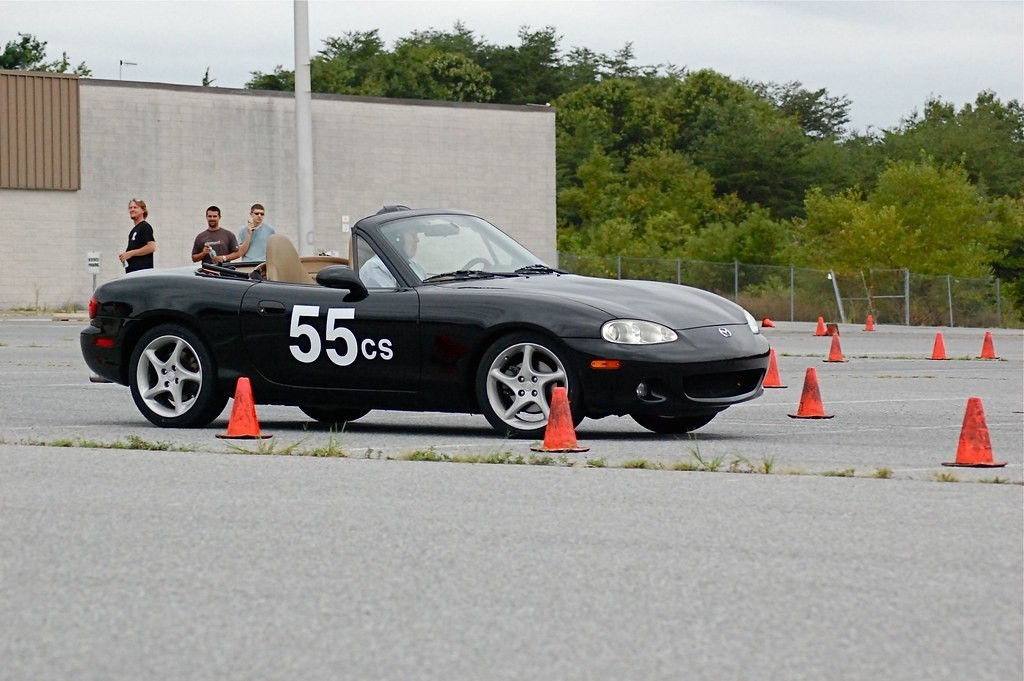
[214,375,274,440]
[761,348,790,389]
[813,316,829,336]
[925,332,951,361]
[940,397,1007,467]
[975,331,1001,360]
[862,314,875,331]
[824,322,840,336]
[785,367,835,419]
[529,385,591,452]
[822,331,849,363]
[761,316,776,328]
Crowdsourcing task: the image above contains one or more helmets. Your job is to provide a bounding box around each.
[376,204,429,246]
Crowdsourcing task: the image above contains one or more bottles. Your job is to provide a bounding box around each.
[119,250,128,268]
[209,247,216,259]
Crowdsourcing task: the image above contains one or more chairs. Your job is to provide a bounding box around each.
[265,234,317,284]
[348,234,373,271]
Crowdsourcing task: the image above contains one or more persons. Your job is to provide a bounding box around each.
[236,204,276,262]
[119,199,156,274]
[359,205,430,287]
[192,206,241,268]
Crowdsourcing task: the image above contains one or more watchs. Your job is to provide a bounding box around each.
[223,255,226,262]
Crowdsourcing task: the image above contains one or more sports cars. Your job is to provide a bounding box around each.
[81,204,771,440]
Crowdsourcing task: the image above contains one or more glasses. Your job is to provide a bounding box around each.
[252,212,264,215]
